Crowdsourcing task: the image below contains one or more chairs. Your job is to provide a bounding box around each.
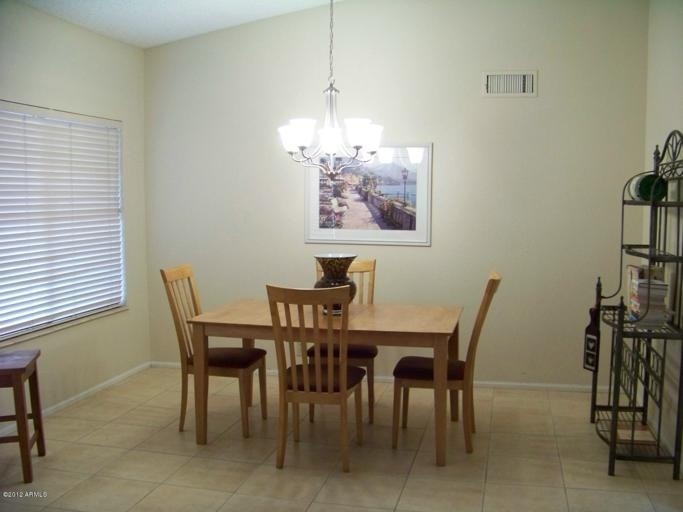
[1,349,45,483]
[160,264,268,438]
[392,268,503,453]
[307,257,376,427]
[266,284,366,474]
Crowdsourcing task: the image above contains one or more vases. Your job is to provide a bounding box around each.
[314,255,358,316]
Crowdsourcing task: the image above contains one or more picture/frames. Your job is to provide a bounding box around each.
[303,142,433,247]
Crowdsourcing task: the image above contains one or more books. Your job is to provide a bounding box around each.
[628,278,669,329]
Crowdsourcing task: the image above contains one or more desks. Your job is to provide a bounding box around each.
[185,300,464,467]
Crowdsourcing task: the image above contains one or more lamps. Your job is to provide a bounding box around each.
[361,145,426,166]
[277,0,383,177]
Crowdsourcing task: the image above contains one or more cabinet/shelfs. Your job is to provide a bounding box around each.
[584,130,683,481]
